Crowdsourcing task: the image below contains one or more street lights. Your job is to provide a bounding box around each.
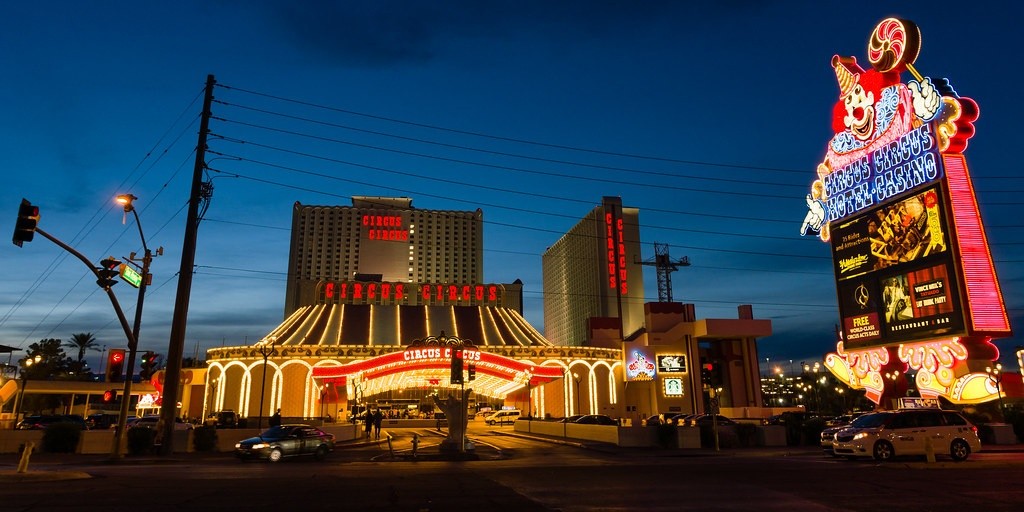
[208,377,219,414]
[985,363,1008,424]
[319,383,329,418]
[573,372,583,417]
[524,366,535,420]
[108,192,164,460]
[257,336,276,429]
[12,354,42,430]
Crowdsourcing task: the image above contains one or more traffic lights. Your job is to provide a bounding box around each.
[105,348,126,384]
[11,197,39,248]
[102,390,117,405]
[145,350,160,380]
[140,354,147,380]
[97,256,123,288]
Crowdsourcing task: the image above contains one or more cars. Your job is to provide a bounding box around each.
[16,416,42,429]
[85,414,216,453]
[644,412,740,428]
[575,414,615,424]
[235,423,337,467]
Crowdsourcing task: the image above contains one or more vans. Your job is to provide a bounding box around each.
[485,409,522,425]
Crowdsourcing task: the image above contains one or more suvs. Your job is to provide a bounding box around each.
[204,410,238,429]
[819,407,982,464]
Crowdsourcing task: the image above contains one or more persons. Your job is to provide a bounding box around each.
[365,407,373,438]
[373,407,382,438]
[884,278,909,320]
[271,408,282,425]
[384,409,416,419]
